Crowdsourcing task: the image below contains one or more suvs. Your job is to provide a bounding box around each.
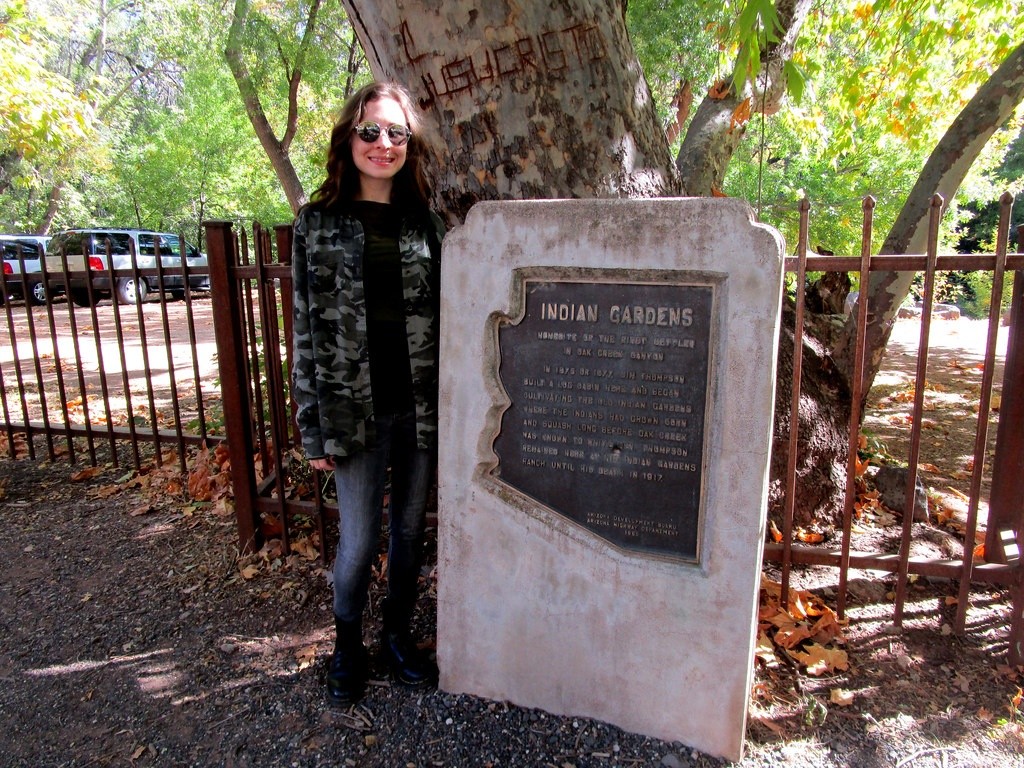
[0,233,54,308]
[44,227,210,309]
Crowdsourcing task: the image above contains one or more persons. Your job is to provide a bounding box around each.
[290,81,446,708]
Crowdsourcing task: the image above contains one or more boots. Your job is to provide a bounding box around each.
[382,599,434,686]
[326,614,369,698]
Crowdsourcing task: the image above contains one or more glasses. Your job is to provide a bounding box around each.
[353,121,413,146]
